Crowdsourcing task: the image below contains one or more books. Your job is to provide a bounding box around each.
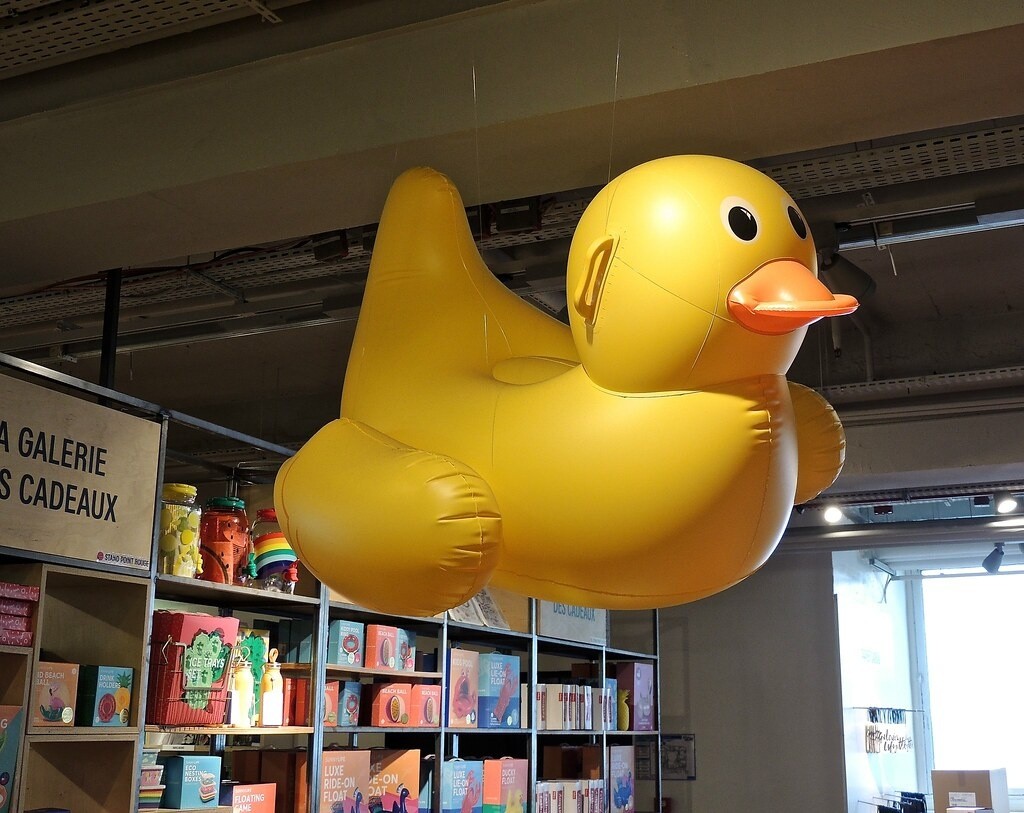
[516,683,613,730]
[535,778,604,813]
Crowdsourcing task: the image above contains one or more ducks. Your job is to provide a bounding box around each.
[273,153,860,618]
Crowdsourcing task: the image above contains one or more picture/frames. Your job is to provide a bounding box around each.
[635,733,696,780]
[537,599,607,647]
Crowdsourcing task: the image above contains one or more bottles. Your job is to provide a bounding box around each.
[157,483,201,579]
[258,648,284,728]
[198,497,251,587]
[233,646,254,728]
[251,508,297,595]
[617,689,630,730]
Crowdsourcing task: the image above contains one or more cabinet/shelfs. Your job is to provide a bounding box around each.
[0,352,663,813]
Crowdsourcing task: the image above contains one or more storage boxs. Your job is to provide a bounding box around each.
[370,683,412,728]
[33,661,133,727]
[146,608,270,725]
[219,780,276,813]
[141,765,164,785]
[283,677,362,726]
[572,662,655,731]
[0,582,40,646]
[138,785,166,809]
[142,748,160,766]
[411,684,442,727]
[158,755,222,808]
[561,745,635,813]
[252,618,416,672]
[930,767,1010,813]
[0,705,23,813]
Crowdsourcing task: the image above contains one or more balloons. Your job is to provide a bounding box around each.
[272,153,859,619]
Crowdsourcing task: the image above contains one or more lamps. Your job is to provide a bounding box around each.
[817,248,877,306]
[869,558,896,577]
[993,491,1018,514]
[981,542,1005,574]
